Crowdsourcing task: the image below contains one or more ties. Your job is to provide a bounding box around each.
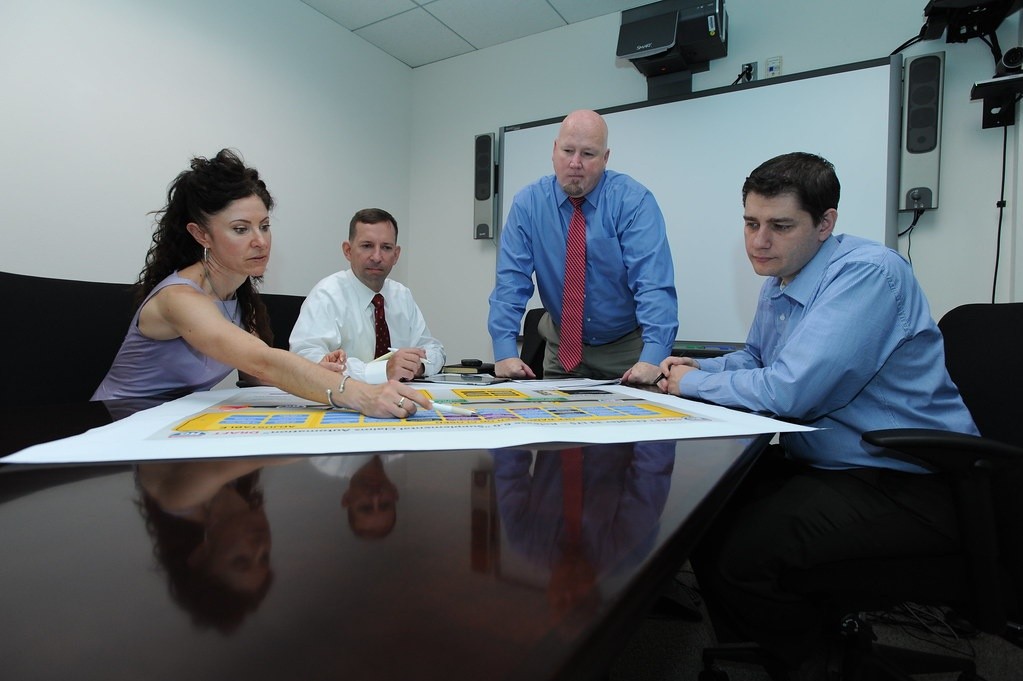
[561,448,584,564]
[558,195,586,373]
[372,294,391,360]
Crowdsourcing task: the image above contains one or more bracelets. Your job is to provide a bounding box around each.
[327,375,351,408]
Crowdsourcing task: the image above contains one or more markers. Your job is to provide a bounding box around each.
[686,345,704,349]
[707,345,734,350]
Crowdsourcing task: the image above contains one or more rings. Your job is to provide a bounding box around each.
[398,396,406,407]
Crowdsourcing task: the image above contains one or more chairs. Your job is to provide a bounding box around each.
[236,292,307,388]
[0,271,141,461]
[700,302,1023,681]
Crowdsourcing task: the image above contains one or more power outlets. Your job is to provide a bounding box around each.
[742,62,758,82]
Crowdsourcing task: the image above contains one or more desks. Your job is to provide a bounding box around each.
[0,379,775,681]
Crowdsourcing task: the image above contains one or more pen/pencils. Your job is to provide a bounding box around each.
[653,362,673,385]
[412,399,473,417]
[388,347,432,367]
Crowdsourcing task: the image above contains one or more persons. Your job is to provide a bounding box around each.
[136,457,274,636]
[655,151,993,680]
[89,148,434,420]
[488,109,679,384]
[306,452,406,538]
[490,439,677,634]
[290,208,446,384]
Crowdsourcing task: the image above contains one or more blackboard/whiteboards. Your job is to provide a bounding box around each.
[476,53,904,358]
